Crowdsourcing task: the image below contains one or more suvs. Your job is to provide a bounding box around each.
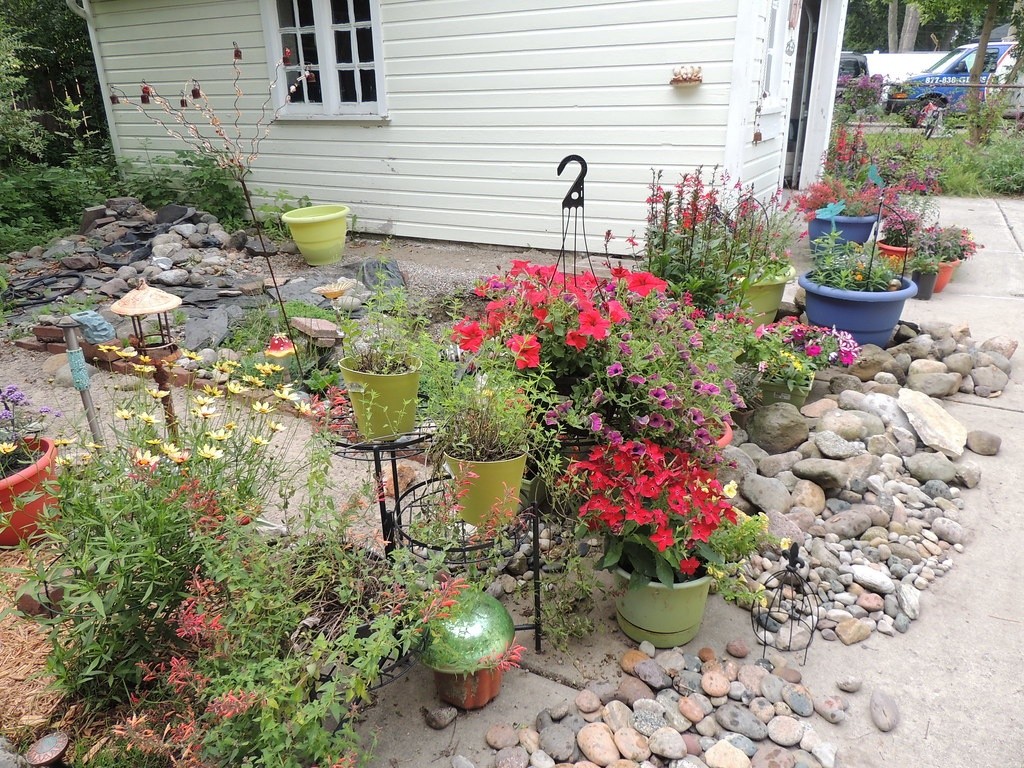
[833,51,870,125]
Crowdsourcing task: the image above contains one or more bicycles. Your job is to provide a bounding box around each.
[919,104,947,141]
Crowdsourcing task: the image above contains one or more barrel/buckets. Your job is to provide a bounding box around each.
[280,205,351,266]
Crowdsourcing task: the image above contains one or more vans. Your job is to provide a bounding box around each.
[882,41,1024,130]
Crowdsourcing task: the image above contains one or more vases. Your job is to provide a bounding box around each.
[878,238,916,272]
[800,268,919,351]
[759,378,814,412]
[806,210,879,257]
[731,273,787,332]
[912,269,938,301]
[0,436,63,549]
[521,462,551,509]
[698,422,734,473]
[609,566,712,649]
[281,204,349,266]
[930,257,960,293]
[557,439,596,471]
[949,258,965,283]
[436,665,503,709]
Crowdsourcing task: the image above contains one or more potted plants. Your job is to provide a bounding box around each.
[334,238,425,440]
[419,287,557,524]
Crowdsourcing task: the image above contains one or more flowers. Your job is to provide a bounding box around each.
[0,384,61,477]
[454,119,982,586]
